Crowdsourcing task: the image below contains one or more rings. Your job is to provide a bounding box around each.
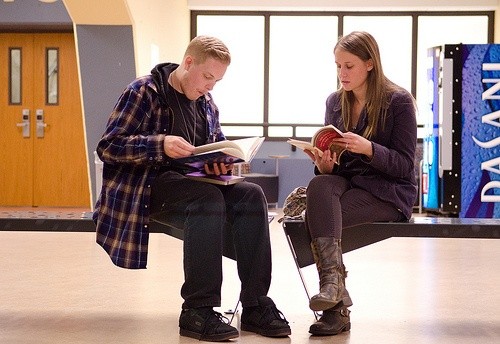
[345,144,348,150]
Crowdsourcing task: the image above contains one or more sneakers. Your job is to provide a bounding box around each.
[179,309,239,341]
[241,297,291,336]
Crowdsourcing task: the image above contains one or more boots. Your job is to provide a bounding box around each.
[309,237,353,335]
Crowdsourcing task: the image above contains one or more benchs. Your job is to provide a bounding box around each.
[0,210,274,324]
[282,209,500,322]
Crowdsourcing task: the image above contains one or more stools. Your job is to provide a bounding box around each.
[241,173,279,209]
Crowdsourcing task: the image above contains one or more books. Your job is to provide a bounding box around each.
[287,124,348,166]
[191,135,266,164]
[186,171,247,186]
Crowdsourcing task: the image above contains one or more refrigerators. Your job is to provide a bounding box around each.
[421,43,500,219]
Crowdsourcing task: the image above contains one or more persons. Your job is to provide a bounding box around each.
[305,30,418,337]
[92,35,292,341]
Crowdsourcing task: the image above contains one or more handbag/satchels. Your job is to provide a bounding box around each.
[278,186,307,223]
